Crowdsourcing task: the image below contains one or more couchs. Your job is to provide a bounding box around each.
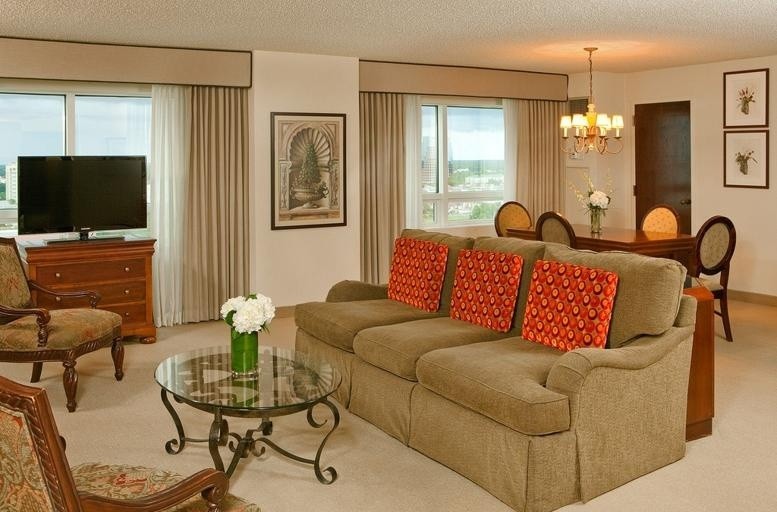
[294,228,696,512]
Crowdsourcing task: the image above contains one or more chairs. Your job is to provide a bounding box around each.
[1,376,260,512]
[1,237,125,411]
[494,201,531,237]
[639,203,682,235]
[690,215,735,343]
[536,211,576,249]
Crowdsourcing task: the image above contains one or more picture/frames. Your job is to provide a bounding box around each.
[722,130,769,189]
[269,111,345,231]
[722,68,769,128]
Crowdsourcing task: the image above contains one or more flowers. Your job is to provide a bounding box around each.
[220,291,276,335]
[568,173,615,209]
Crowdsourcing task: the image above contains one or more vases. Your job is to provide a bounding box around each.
[230,330,260,376]
[589,210,601,234]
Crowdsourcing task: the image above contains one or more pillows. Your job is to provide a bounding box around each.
[448,248,523,333]
[521,259,618,352]
[387,238,448,313]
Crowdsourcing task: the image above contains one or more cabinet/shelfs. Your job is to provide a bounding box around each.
[679,285,715,442]
[17,235,157,344]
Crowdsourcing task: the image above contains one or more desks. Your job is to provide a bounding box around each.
[504,223,697,280]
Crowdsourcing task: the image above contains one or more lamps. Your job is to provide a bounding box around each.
[558,46,623,157]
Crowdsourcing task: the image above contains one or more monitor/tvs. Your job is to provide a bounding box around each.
[17,156,147,245]
